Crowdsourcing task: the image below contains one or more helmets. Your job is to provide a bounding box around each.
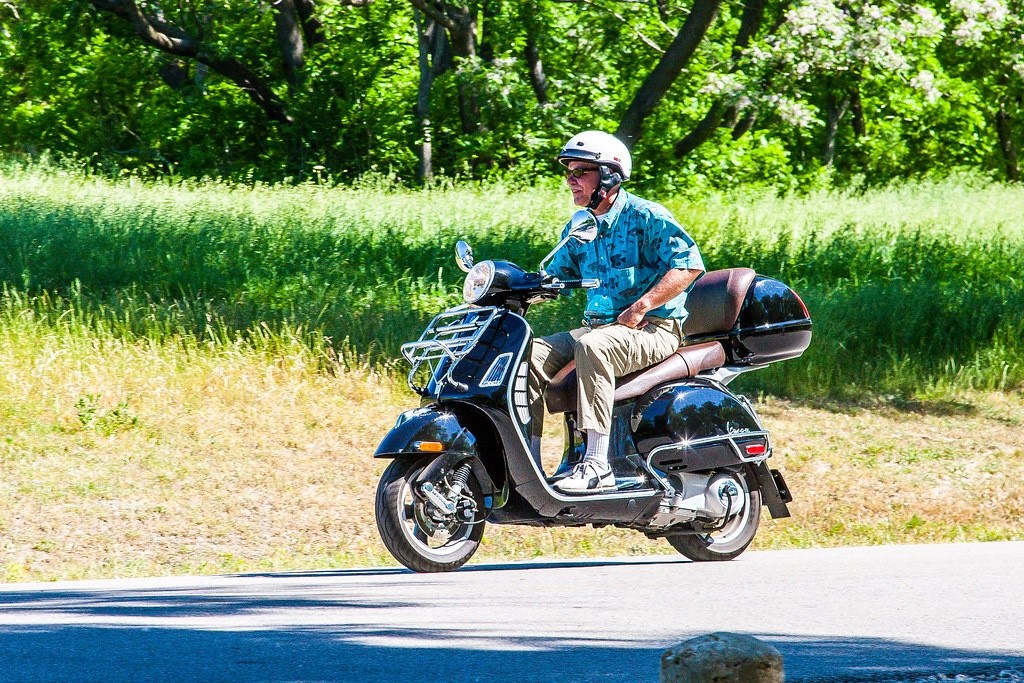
[557,130,632,182]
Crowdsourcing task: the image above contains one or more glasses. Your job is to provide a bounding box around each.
[566,168,599,178]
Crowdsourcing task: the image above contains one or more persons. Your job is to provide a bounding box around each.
[528,130,706,496]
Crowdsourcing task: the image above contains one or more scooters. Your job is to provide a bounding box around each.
[373,238,812,572]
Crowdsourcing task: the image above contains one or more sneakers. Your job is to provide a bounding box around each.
[553,461,618,494]
